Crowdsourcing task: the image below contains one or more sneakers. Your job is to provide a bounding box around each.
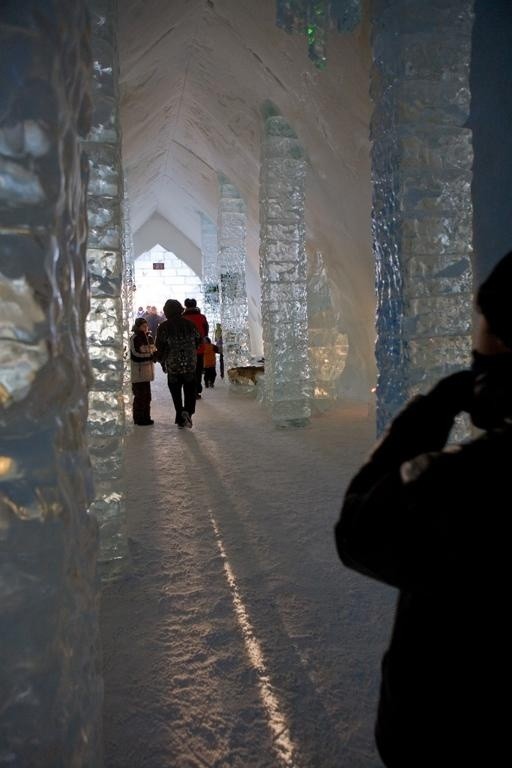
[176,410,193,429]
[196,383,215,400]
[133,419,154,426]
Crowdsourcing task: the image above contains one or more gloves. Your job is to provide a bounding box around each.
[423,372,472,418]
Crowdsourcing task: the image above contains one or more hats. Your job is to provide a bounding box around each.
[478,250,512,347]
[184,298,196,308]
[135,318,146,327]
[163,299,184,318]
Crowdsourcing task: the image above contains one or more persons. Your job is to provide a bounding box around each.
[130,298,224,429]
[334,249,512,768]
